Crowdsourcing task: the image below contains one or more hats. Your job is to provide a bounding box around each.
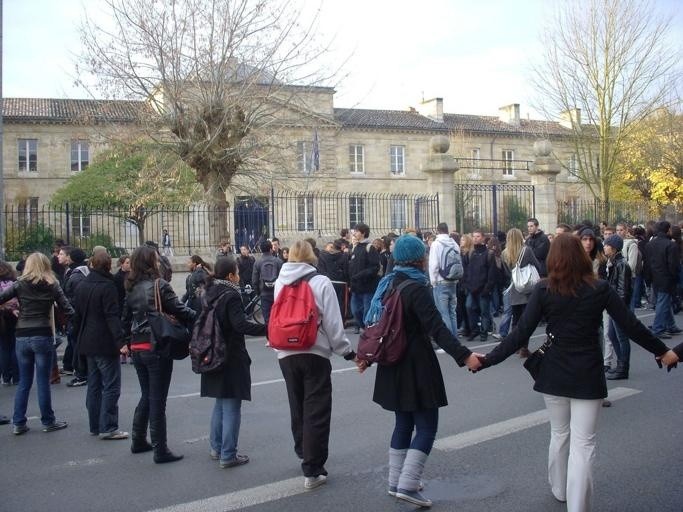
[604,234,624,252]
[392,234,426,264]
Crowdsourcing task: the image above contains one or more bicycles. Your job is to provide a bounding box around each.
[236,287,264,325]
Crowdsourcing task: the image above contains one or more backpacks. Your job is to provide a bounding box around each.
[636,249,645,277]
[356,287,408,367]
[501,260,511,289]
[261,259,278,291]
[189,305,229,376]
[147,309,190,360]
[266,278,319,352]
[376,260,384,278]
[440,245,465,281]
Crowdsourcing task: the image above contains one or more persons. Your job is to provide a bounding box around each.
[305,224,417,334]
[557,221,683,379]
[418,219,554,357]
[0,242,212,463]
[202,259,267,468]
[274,240,364,489]
[215,226,289,346]
[162,229,172,256]
[468,233,682,512]
[357,234,486,505]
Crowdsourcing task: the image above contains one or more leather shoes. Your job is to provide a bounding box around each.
[479,333,488,342]
[607,373,629,380]
[130,443,153,454]
[664,326,683,334]
[607,367,629,373]
[466,331,481,342]
[657,333,672,340]
[210,447,221,461]
[219,454,250,470]
[153,452,185,463]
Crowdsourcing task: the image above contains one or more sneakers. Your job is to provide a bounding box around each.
[42,421,67,433]
[101,429,130,440]
[303,475,327,489]
[491,333,504,341]
[388,480,425,497]
[13,424,30,436]
[67,378,87,388]
[396,487,433,507]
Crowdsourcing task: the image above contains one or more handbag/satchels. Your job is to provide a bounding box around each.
[62,344,76,372]
[512,264,541,294]
[524,344,549,381]
